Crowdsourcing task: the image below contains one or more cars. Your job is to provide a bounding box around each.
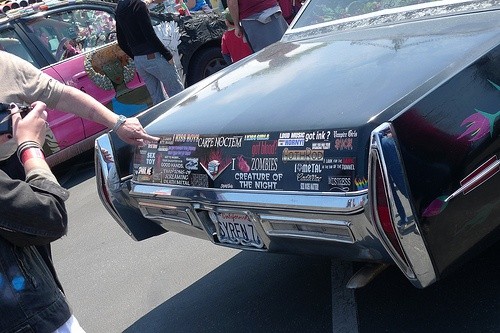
[94,0,500,296]
[0,1,236,183]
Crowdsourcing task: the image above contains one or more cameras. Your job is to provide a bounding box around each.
[0,103,33,136]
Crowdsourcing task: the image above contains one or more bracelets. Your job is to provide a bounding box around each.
[16,140,45,167]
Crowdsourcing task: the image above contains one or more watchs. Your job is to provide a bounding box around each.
[113,115,126,133]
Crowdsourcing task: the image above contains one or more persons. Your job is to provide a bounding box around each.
[116,0,184,106]
[227,0,289,53]
[0,50,160,261]
[0,101,87,333]
[182,0,210,11]
[221,8,254,65]
